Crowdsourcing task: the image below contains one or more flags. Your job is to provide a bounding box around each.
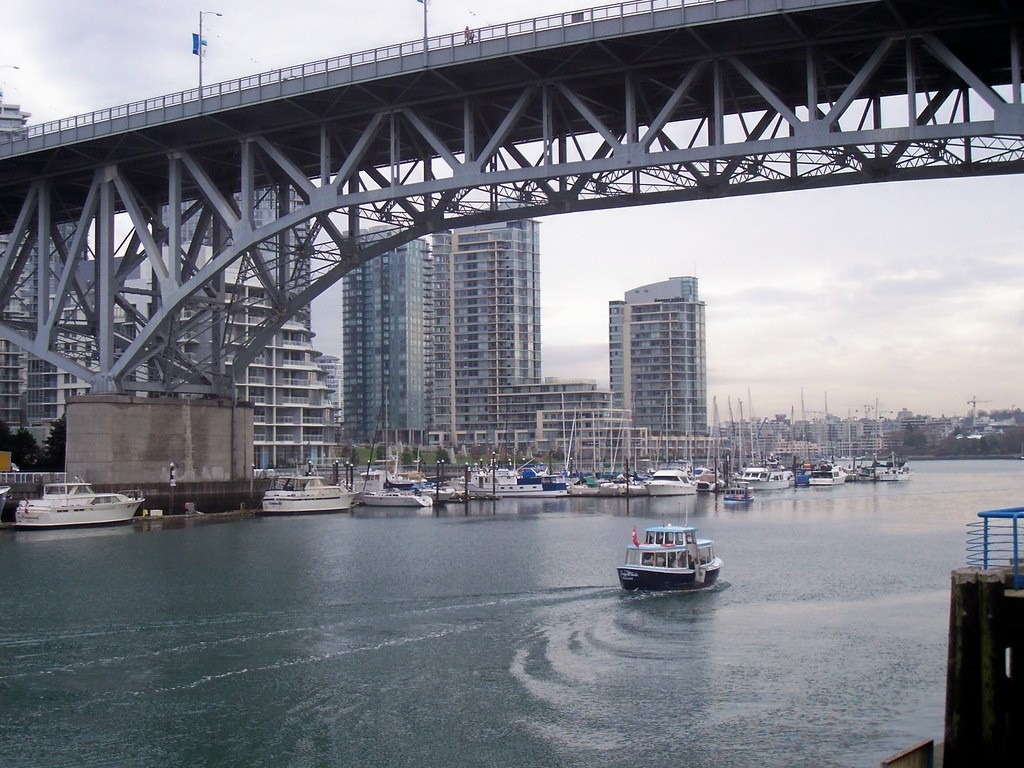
[134,490,138,501]
[632,527,639,548]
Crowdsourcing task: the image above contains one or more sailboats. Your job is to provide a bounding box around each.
[261,392,915,512]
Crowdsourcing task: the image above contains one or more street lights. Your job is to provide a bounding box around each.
[198,11,225,87]
[0,66,22,109]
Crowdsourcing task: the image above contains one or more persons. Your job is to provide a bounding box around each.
[680,550,692,567]
[464,26,472,43]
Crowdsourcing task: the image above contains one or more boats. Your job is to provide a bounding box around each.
[15,476,145,524]
[617,525,724,590]
